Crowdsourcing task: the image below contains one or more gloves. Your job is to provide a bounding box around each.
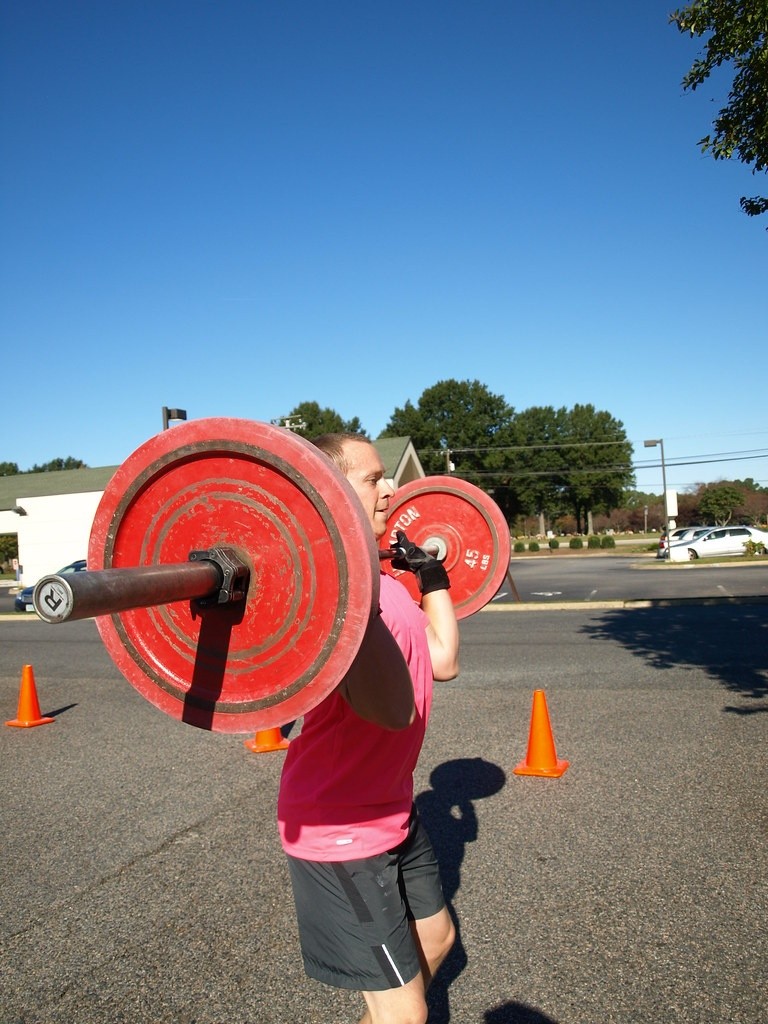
[389,530,451,595]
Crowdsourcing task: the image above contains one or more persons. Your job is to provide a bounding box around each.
[277,434,460,1024]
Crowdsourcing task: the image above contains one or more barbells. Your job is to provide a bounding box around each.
[30,413,513,735]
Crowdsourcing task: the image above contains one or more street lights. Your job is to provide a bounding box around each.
[644,438,671,563]
[160,407,190,435]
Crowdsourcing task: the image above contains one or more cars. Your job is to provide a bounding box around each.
[656,525,768,560]
[14,558,90,616]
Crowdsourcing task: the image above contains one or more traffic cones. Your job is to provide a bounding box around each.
[3,664,57,729]
[240,724,292,756]
[513,689,571,781]
